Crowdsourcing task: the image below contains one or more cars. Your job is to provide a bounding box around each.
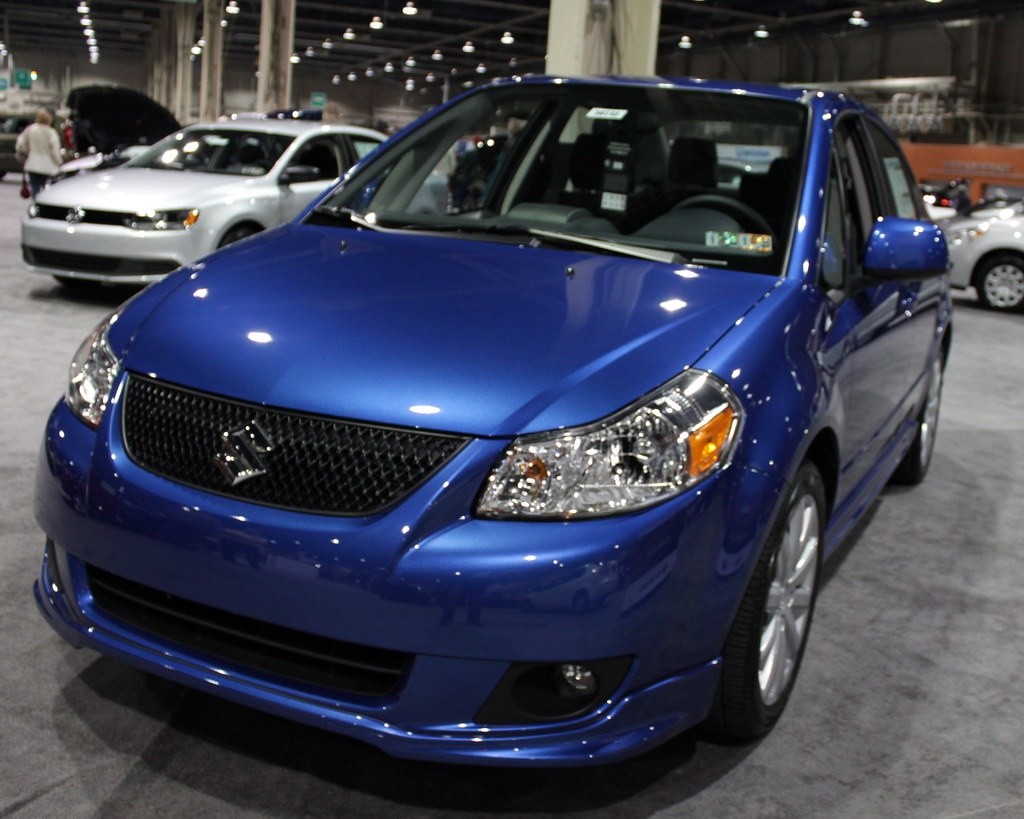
[34,73,956,764]
[46,131,267,188]
[20,118,449,291]
[0,110,89,179]
[918,180,1024,312]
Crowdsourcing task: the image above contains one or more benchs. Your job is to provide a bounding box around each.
[616,138,799,234]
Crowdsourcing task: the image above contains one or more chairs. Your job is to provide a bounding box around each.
[507,201,589,224]
[239,146,266,163]
[309,144,334,174]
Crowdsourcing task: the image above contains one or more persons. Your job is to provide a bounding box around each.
[15,109,63,199]
